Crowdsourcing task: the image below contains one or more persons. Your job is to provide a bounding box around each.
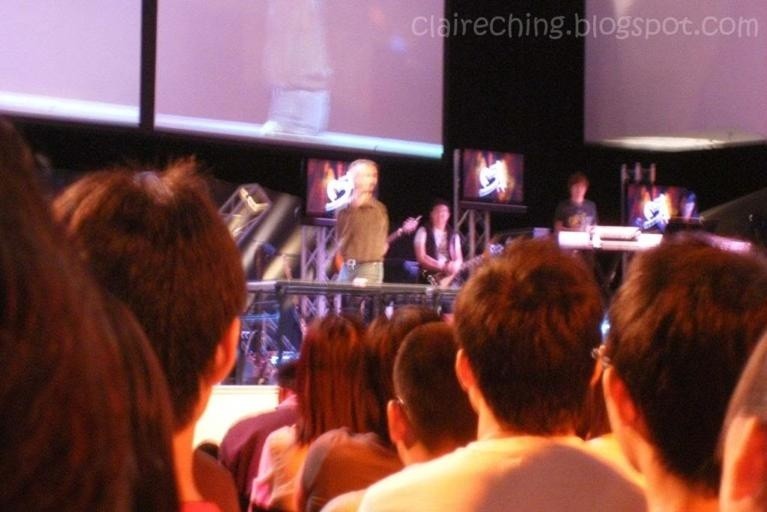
[1,117,767,510]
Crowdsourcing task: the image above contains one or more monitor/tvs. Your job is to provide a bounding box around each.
[299,155,351,226]
[455,143,530,214]
[624,173,696,231]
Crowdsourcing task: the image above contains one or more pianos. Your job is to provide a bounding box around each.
[534,225,664,251]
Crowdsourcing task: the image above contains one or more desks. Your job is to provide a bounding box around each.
[235,280,460,386]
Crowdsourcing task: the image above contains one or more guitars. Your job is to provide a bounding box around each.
[423,245,502,289]
